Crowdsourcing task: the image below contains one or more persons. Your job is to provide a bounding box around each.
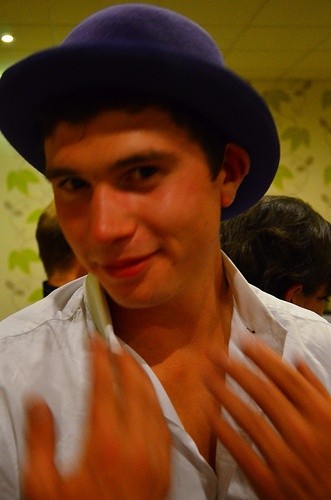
[220,195,331,314]
[0,3,331,500]
[35,198,87,297]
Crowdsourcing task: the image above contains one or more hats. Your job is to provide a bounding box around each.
[0,4,281,221]
